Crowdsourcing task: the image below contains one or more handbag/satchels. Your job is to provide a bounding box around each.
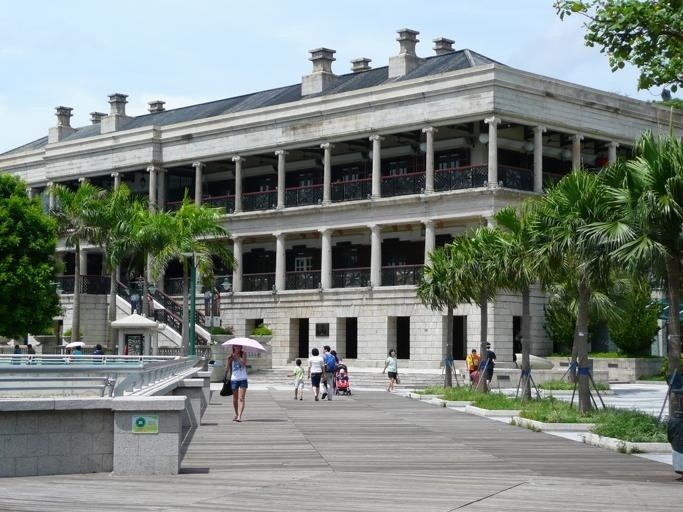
[220,379,232,396]
[396,377,400,385]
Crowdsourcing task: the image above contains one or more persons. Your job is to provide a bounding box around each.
[287,345,352,402]
[465,349,481,385]
[26,344,37,364]
[91,344,106,365]
[11,344,22,364]
[382,348,400,393]
[73,346,83,358]
[486,342,496,391]
[224,344,248,422]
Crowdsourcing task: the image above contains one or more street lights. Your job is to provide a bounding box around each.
[180,249,208,355]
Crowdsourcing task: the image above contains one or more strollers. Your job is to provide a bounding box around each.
[334,364,352,396]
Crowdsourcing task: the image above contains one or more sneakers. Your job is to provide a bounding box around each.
[315,393,327,400]
[233,416,241,422]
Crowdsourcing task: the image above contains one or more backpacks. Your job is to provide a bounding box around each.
[325,353,335,372]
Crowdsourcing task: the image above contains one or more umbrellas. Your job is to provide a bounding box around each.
[66,342,85,347]
[649,297,683,320]
[221,337,267,352]
